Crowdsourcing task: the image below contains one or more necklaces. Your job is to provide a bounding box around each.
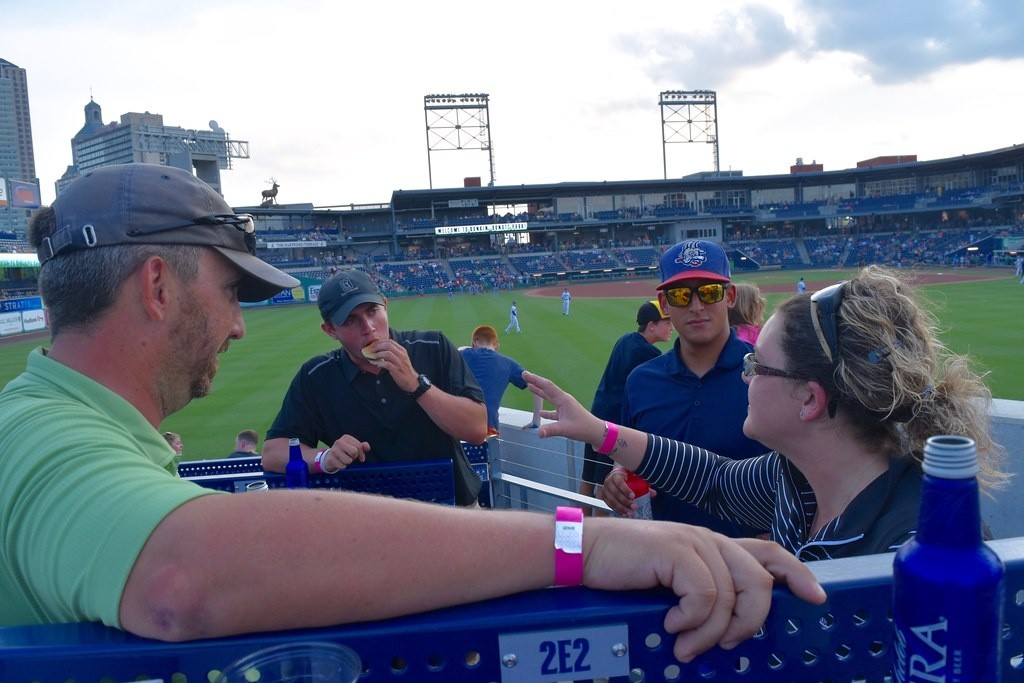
[809,510,819,536]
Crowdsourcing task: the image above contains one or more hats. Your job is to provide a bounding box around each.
[38,163,301,303]
[654,239,731,291]
[318,271,385,326]
[637,301,670,325]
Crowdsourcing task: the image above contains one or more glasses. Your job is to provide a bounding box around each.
[743,353,818,383]
[809,272,844,418]
[126,212,256,255]
[662,283,727,308]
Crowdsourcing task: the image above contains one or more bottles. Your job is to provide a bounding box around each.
[285,438,311,489]
[625,476,653,520]
[888,436,1004,682]
[247,481,268,492]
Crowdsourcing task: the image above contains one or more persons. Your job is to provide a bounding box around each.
[726,282,766,343]
[227,430,261,458]
[162,432,183,455]
[261,271,488,512]
[0,162,826,662]
[797,278,806,294]
[521,265,1003,563]
[252,184,1024,302]
[458,326,543,431]
[0,288,33,299]
[505,300,522,334]
[561,287,572,316]
[579,300,673,518]
[602,241,775,540]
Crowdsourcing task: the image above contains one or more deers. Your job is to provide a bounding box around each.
[262,177,281,204]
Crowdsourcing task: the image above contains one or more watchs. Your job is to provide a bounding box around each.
[407,374,432,400]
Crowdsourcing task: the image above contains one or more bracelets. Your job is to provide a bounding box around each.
[314,448,340,475]
[591,421,618,455]
[552,507,585,587]
[531,422,540,428]
